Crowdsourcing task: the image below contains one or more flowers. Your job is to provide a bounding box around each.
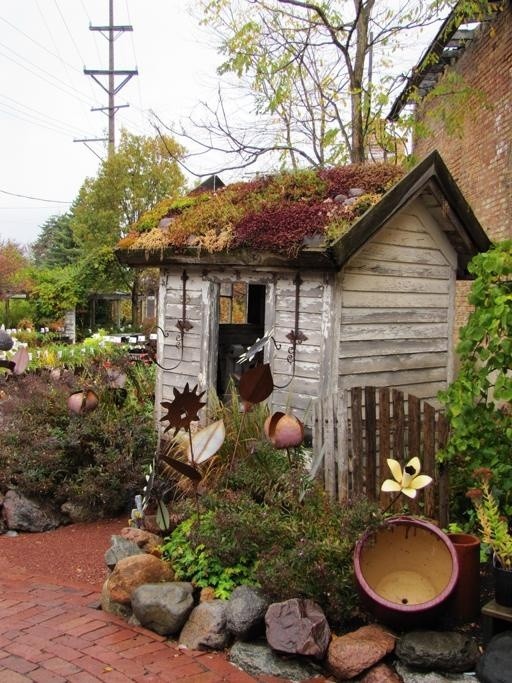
[378,456,433,515]
[466,465,512,568]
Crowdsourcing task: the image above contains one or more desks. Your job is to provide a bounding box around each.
[480,599,512,640]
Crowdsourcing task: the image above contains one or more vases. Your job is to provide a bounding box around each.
[494,565,512,607]
[444,532,479,610]
[354,515,459,621]
